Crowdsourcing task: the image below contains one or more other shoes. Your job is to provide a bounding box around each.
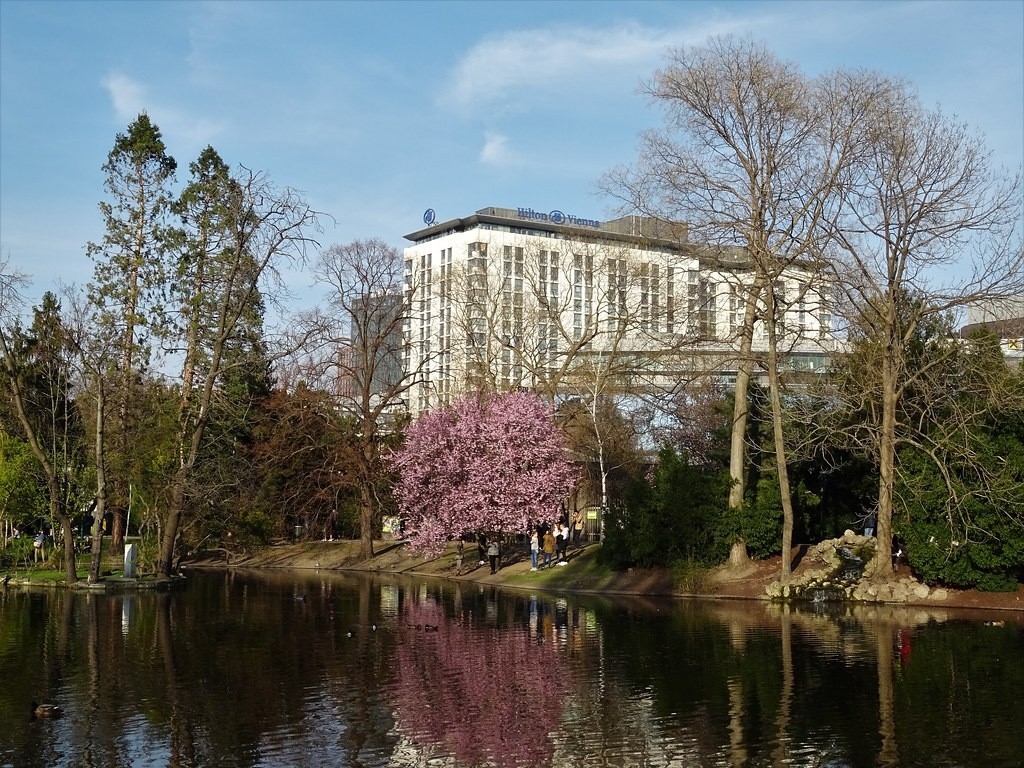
[530,566,537,572]
[479,561,485,565]
[490,571,495,575]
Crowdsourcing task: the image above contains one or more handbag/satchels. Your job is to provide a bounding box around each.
[33,541,42,549]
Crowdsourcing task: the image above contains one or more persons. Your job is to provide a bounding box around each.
[891,528,900,571]
[49,527,92,555]
[486,535,499,574]
[571,510,584,549]
[455,538,466,576]
[12,527,20,539]
[476,529,486,565]
[399,508,410,535]
[529,524,569,571]
[863,506,874,537]
[34,528,47,562]
